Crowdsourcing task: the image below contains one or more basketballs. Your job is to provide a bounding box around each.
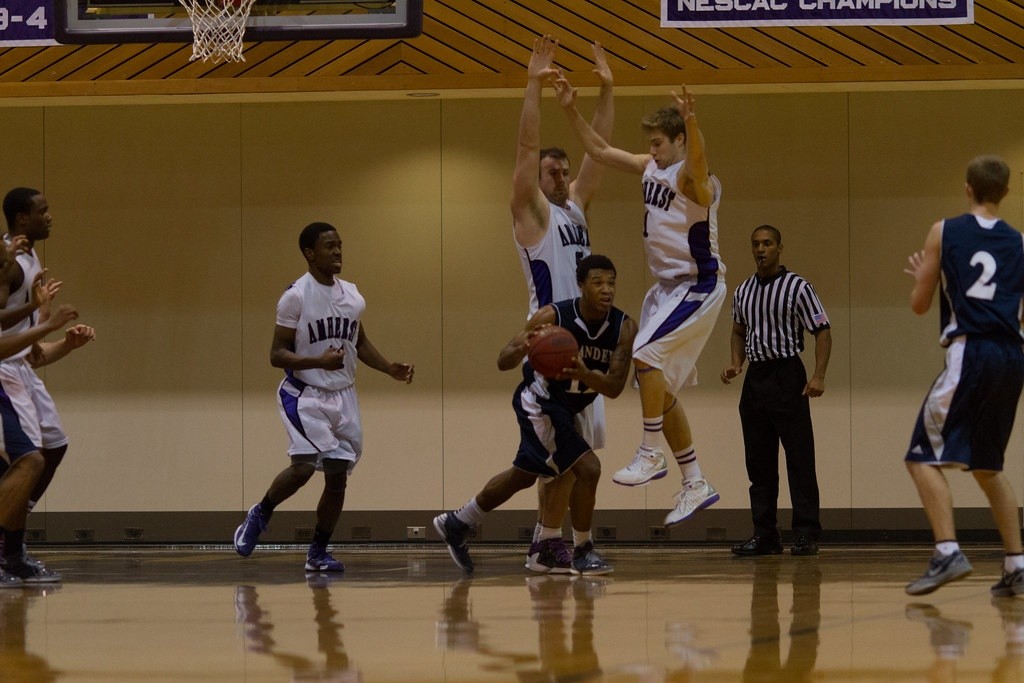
[526,326,578,379]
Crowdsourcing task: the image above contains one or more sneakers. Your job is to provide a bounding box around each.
[664,478,720,526]
[570,576,615,595]
[0,557,24,588]
[613,448,668,487]
[523,540,573,573]
[731,534,784,555]
[570,540,615,575]
[305,542,344,571]
[905,549,973,595]
[992,596,1024,626]
[233,503,273,557]
[791,535,818,555]
[990,562,1024,598]
[5,543,63,583]
[525,574,579,608]
[432,511,473,572]
[905,604,974,652]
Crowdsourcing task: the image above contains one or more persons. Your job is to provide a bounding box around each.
[233,221,414,571]
[0,187,96,584]
[510,34,616,574]
[720,224,833,556]
[548,69,726,528]
[905,155,1024,598]
[433,254,637,576]
